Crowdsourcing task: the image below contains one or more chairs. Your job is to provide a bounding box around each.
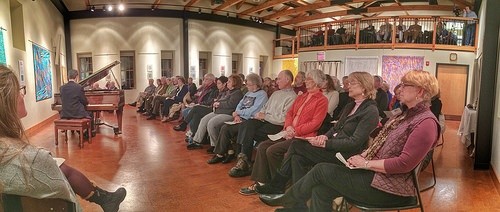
[339,113,446,212]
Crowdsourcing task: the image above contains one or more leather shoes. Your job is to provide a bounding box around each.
[258,193,297,209]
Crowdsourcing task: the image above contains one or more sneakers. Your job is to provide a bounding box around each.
[228,157,252,177]
[239,181,261,196]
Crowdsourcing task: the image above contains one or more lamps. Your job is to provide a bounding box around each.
[249,15,264,23]
[91,5,96,12]
[103,4,105,12]
[198,9,238,18]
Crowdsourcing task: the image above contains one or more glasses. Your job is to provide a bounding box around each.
[19,85,26,96]
[399,83,420,89]
[245,82,257,86]
[347,82,360,87]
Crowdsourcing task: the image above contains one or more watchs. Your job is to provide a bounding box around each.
[364,161,368,167]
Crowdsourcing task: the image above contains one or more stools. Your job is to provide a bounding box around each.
[54,118,92,148]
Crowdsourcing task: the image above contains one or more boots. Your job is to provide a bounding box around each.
[85,185,127,212]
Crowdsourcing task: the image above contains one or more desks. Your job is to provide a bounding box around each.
[460,105,477,155]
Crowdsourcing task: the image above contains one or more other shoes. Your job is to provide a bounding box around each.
[206,155,225,164]
[128,102,210,145]
[187,142,204,149]
[222,152,236,164]
[83,130,88,139]
[255,183,286,194]
[274,204,308,212]
[207,146,215,154]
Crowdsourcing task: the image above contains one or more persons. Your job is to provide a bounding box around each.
[0,63,127,212]
[312,18,452,45]
[60,70,95,140]
[84,82,118,120]
[207,74,268,164]
[128,69,442,212]
[462,6,478,47]
[259,69,439,212]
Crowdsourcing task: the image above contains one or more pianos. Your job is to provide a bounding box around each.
[50,60,125,135]
